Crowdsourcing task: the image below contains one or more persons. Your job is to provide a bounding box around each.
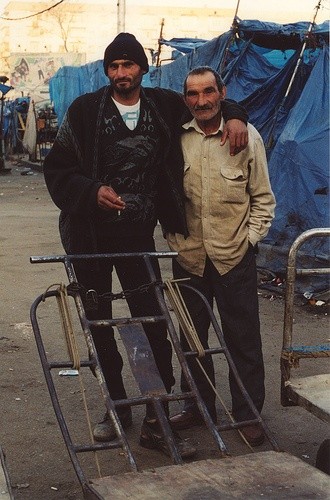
[42,33,248,457]
[162,65,277,446]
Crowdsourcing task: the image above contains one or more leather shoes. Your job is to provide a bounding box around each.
[169,411,217,429]
[240,424,264,446]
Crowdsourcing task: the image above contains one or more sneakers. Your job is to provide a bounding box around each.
[140,417,196,460]
[93,416,132,442]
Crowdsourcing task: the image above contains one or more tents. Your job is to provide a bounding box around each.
[48,0,330,290]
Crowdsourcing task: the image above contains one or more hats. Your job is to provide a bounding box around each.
[103,33,149,77]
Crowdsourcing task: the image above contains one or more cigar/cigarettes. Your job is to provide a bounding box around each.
[117,196,121,216]
